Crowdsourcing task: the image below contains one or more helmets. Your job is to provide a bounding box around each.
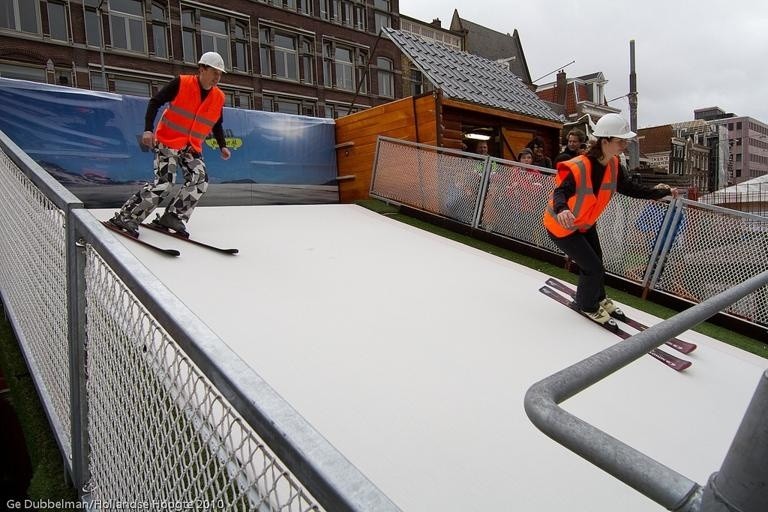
[592,113,637,139]
[198,52,227,73]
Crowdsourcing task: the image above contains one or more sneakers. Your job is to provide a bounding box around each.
[110,213,139,237]
[159,213,189,237]
[582,297,625,328]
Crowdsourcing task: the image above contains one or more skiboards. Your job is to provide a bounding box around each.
[98,211,239,256]
[539,278,696,372]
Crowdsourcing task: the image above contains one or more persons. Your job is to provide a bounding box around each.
[107,51,231,240]
[542,113,679,329]
[448,127,595,250]
